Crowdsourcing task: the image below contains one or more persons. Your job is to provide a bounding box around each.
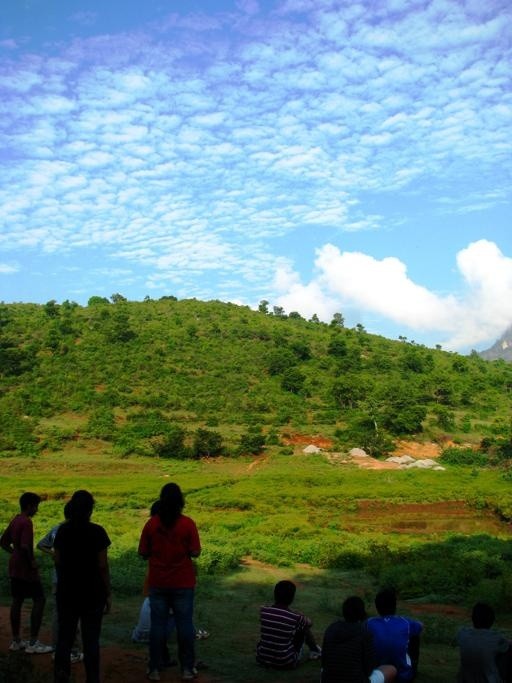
[0,491,53,653]
[52,489,112,683]
[320,595,398,683]
[137,482,201,681]
[149,499,210,640]
[365,590,424,683]
[255,580,321,670]
[455,603,512,683]
[36,501,85,664]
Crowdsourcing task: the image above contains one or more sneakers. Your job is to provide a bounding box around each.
[25,641,54,654]
[146,667,161,681]
[70,652,85,664]
[9,639,28,652]
[195,629,210,641]
[182,668,198,680]
[310,644,322,660]
